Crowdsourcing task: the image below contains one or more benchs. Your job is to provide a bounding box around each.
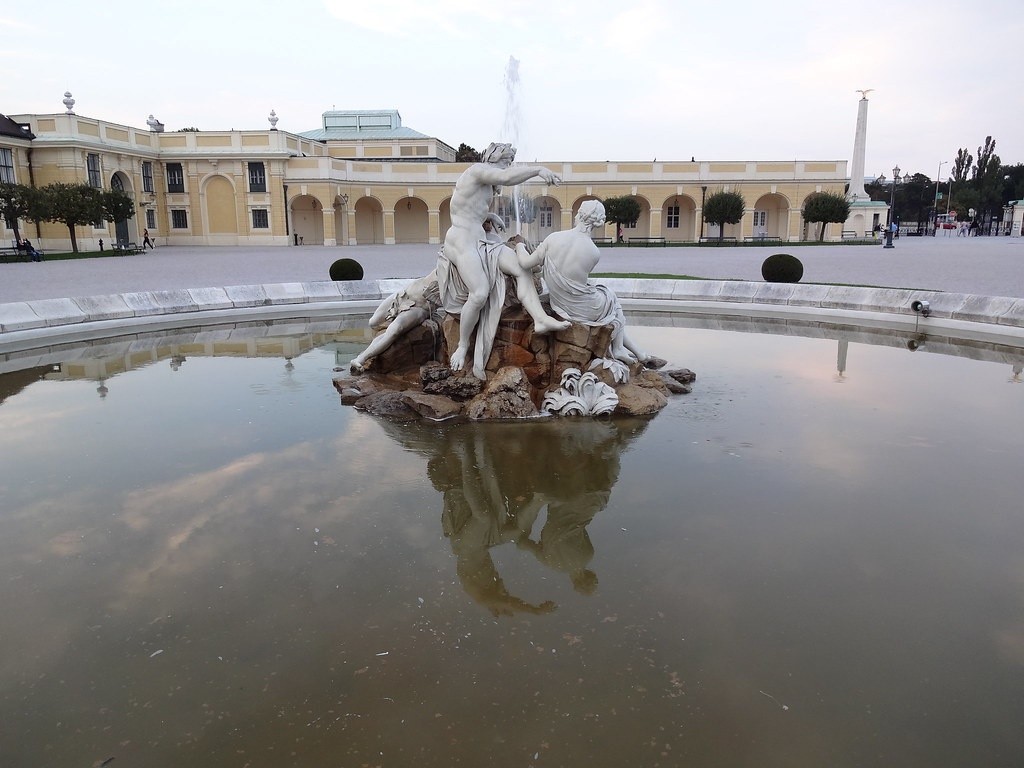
[698,237,737,247]
[742,236,783,246]
[592,237,613,247]
[841,230,857,239]
[0,246,46,264]
[864,231,886,239]
[627,237,665,247]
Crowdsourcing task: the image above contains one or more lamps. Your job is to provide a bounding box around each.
[907,333,927,352]
[311,200,318,208]
[139,192,155,207]
[407,198,411,210]
[911,299,930,318]
[673,196,679,207]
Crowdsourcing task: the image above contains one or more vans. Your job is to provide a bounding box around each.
[935,214,955,227]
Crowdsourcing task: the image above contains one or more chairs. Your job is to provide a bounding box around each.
[110,242,146,257]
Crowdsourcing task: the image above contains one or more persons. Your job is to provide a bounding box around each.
[959,217,985,237]
[617,227,624,244]
[873,223,881,240]
[513,199,652,366]
[349,267,443,373]
[889,222,896,240]
[17,238,42,262]
[143,228,153,250]
[442,141,573,371]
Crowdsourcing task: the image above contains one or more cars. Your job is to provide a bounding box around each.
[936,221,957,229]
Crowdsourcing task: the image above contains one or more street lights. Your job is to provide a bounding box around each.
[934,161,948,207]
[947,177,951,222]
[888,164,900,221]
[701,186,707,237]
